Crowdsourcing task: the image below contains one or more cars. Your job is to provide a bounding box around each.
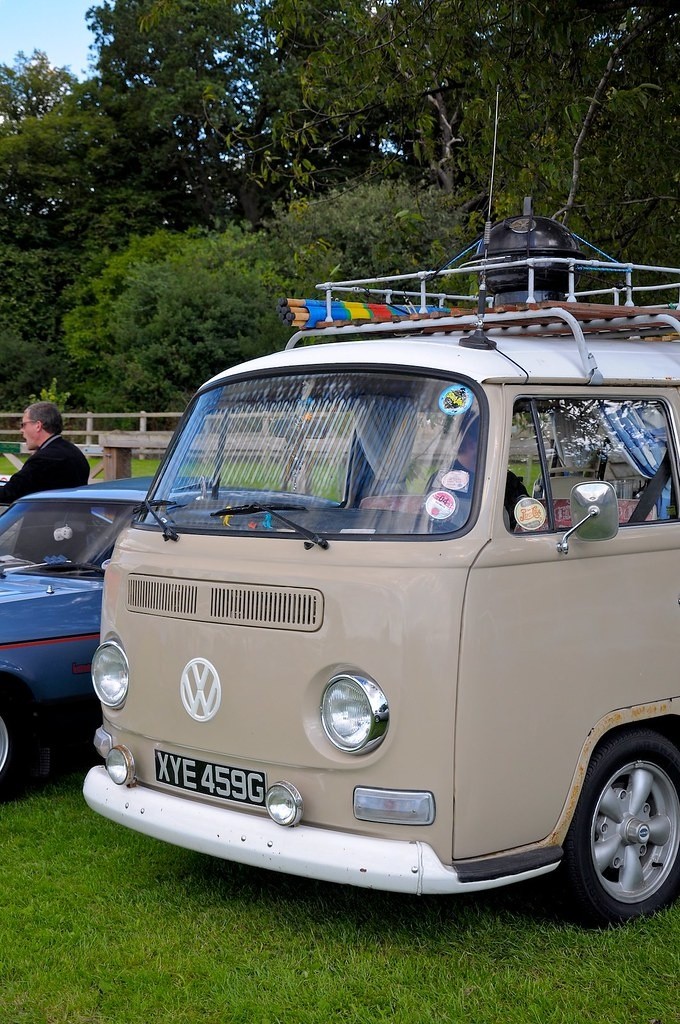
[0,475,352,805]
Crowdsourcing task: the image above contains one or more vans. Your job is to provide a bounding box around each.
[82,193,679,927]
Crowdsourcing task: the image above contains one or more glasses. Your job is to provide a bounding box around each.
[19,420,37,427]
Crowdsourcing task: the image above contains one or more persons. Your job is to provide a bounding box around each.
[414,407,538,533]
[0,401,91,564]
